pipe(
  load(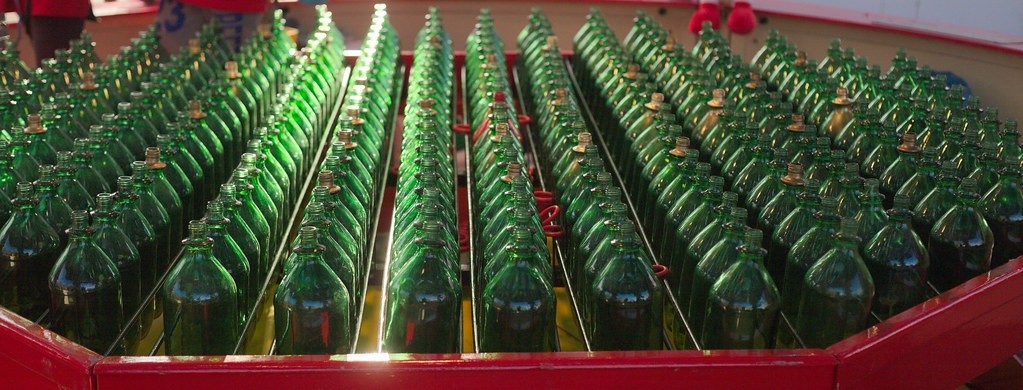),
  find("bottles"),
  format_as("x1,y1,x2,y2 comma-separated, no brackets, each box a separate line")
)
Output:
0,3,1023,357
